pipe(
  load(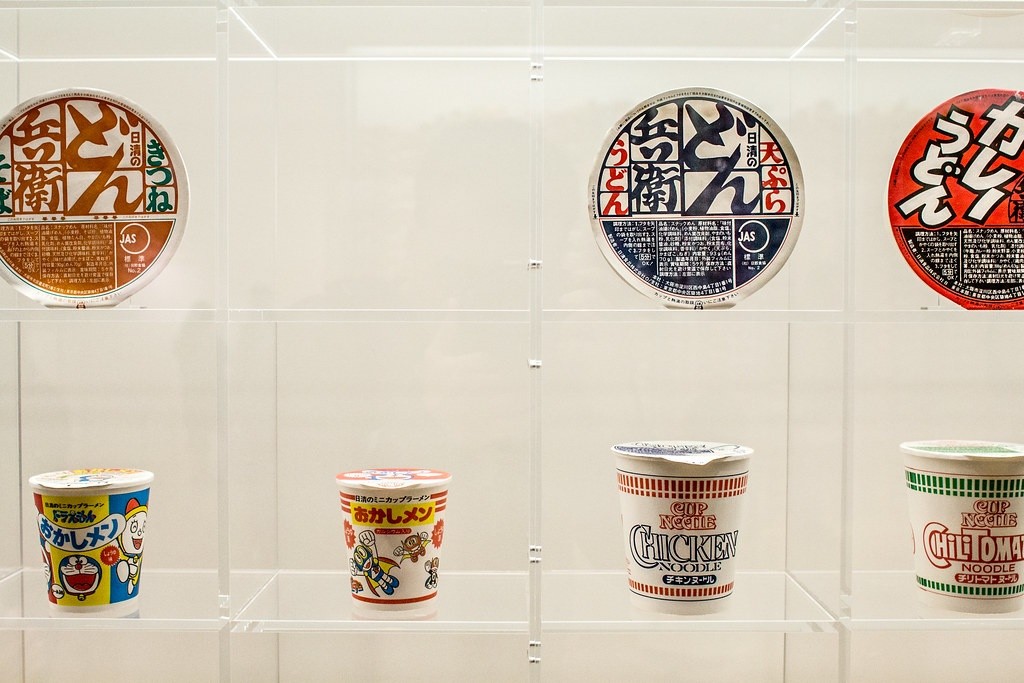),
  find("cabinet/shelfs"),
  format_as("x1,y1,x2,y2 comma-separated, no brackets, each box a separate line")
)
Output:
0,0,1024,682
